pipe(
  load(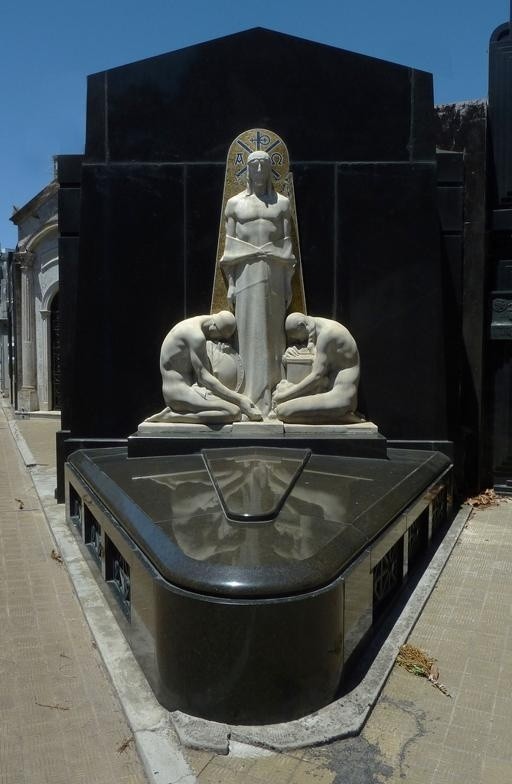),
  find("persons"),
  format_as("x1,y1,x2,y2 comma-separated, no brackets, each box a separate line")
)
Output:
144,310,263,425
219,150,298,422
274,312,368,423
